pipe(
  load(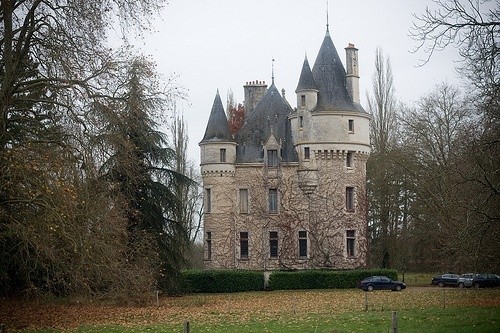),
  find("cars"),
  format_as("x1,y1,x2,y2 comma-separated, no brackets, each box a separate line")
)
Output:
357,276,406,291
457,273,481,288
432,273,461,287
472,274,500,288
